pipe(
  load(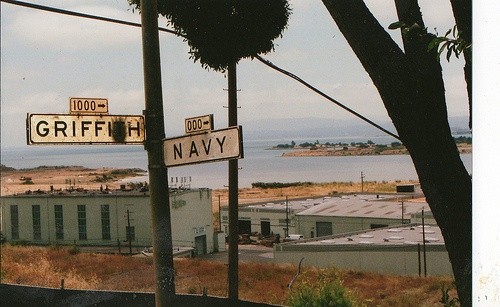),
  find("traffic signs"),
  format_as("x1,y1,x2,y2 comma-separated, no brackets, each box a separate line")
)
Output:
26,96,243,169
